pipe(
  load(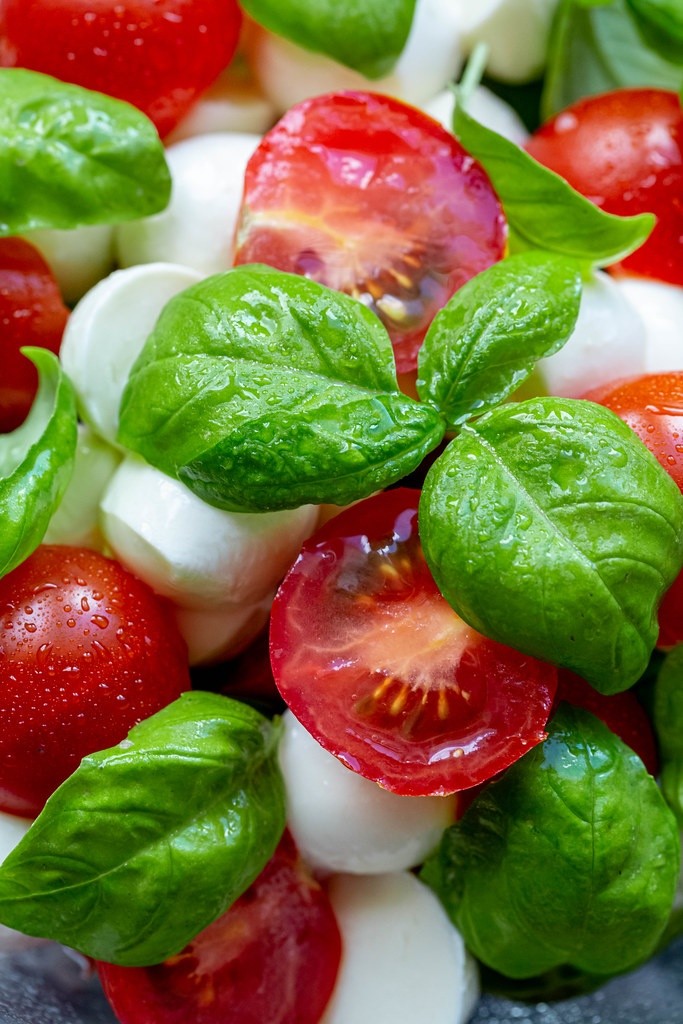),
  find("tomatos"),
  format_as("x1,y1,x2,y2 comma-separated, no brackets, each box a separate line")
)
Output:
0,0,683,1024
